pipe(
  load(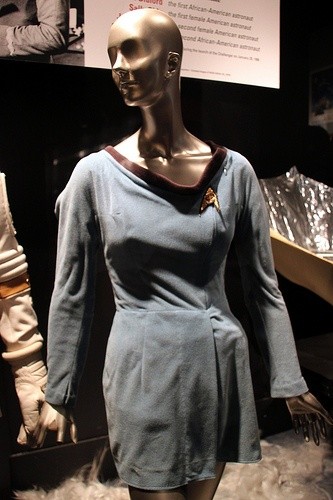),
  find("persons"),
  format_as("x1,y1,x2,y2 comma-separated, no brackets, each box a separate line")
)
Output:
0,0,70,64
31,8,333,500
0,171,47,445
